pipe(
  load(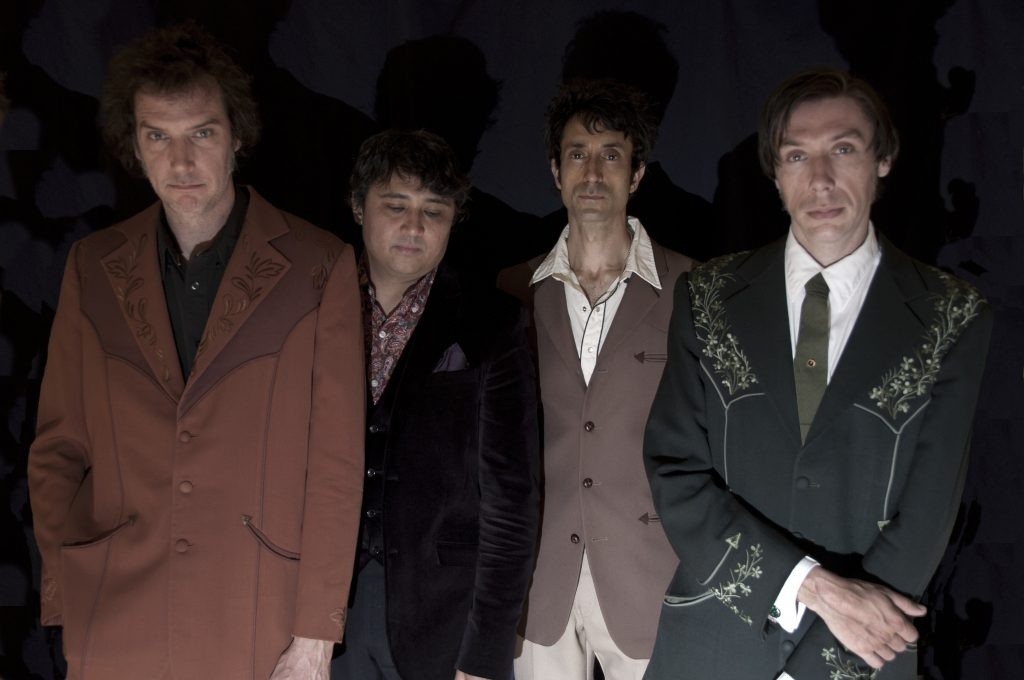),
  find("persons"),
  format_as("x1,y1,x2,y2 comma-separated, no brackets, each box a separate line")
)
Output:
329,127,544,680
497,85,703,679
28,32,365,680
643,69,993,679
